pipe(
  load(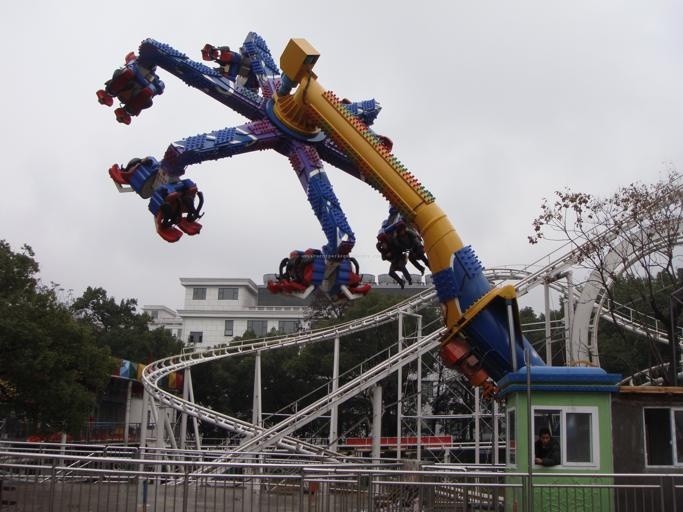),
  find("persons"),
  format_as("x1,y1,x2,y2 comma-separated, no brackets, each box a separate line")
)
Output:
535,428,560,466
397,223,431,276
380,241,412,289
